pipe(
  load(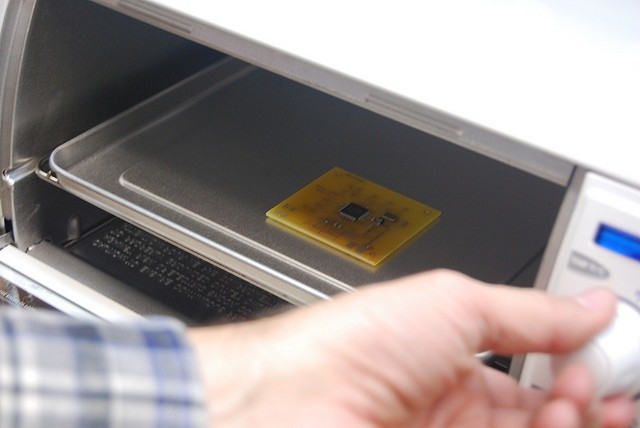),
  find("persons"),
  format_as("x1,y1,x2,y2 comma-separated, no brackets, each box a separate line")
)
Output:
1,268,636,426
